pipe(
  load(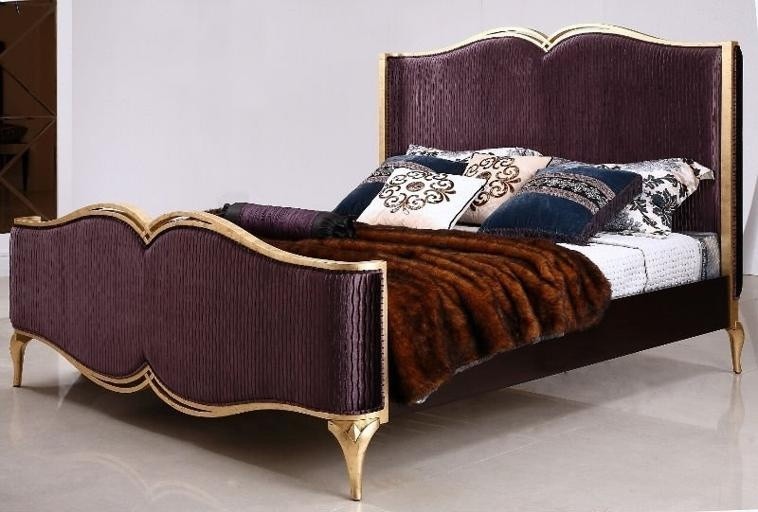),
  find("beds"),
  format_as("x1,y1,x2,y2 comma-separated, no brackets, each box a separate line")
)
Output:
11,23,746,502
